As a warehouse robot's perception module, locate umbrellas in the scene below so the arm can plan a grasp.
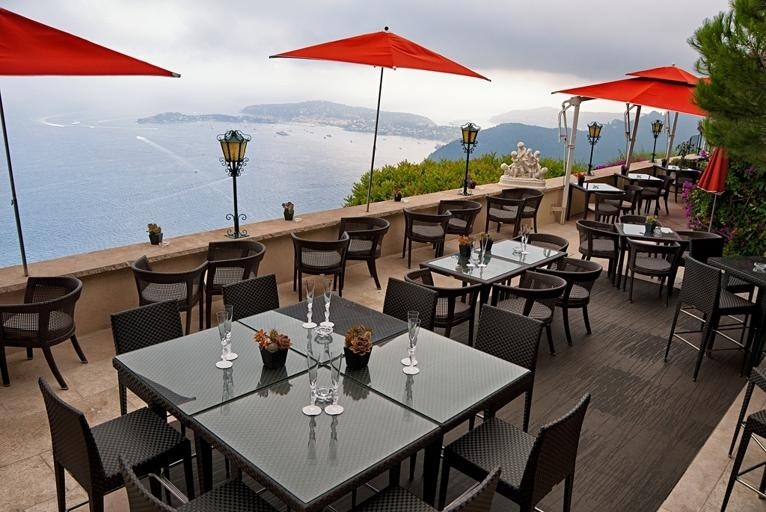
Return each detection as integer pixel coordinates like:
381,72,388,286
266,24,492,212
548,62,733,233
0,7,181,277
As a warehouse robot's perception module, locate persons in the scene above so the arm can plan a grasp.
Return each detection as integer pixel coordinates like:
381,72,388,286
500,141,548,182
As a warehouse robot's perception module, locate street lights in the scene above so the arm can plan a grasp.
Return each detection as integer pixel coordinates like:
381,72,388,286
584,118,604,179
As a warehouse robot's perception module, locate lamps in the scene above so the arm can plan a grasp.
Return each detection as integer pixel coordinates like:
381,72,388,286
583,120,603,175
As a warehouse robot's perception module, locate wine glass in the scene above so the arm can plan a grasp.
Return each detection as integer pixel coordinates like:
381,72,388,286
302,350,322,418
302,278,318,329
326,351,344,416
399,310,418,367
403,318,421,375
320,280,335,328
218,305,238,361
466,234,489,268
520,222,531,255
215,310,233,369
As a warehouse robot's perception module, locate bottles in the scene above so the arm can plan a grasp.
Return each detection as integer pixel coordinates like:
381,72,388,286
653,226,663,238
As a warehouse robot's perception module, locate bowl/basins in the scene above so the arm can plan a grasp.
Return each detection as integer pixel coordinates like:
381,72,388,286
754,262,766,271
